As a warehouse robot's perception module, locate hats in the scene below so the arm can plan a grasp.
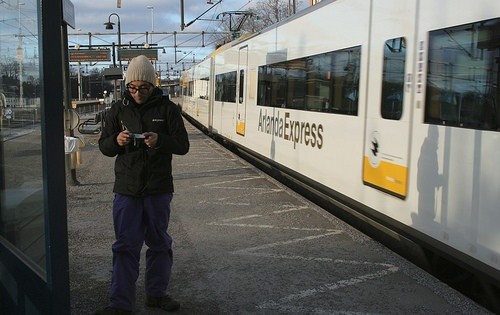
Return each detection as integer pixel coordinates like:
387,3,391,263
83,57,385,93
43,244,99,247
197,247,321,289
125,55,156,90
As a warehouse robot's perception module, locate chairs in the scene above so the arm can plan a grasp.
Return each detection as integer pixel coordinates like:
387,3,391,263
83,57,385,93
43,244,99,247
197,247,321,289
78,107,110,134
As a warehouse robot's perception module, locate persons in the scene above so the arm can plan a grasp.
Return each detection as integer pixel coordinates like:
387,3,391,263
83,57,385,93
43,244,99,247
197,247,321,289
94,55,190,315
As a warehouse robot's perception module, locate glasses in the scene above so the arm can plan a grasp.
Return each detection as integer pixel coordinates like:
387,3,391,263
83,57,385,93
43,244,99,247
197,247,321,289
127,87,151,94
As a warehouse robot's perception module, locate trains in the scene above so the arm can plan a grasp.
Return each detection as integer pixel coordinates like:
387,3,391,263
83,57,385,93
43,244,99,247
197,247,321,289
178,0,500,292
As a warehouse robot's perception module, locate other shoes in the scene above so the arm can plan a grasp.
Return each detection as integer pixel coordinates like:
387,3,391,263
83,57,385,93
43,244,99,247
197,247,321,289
91,304,134,315
145,295,181,311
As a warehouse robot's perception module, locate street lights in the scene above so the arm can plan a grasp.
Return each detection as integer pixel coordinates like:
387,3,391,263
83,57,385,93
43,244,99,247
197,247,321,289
18,2,25,107
145,5,155,45
103,13,124,99
77,28,83,101
